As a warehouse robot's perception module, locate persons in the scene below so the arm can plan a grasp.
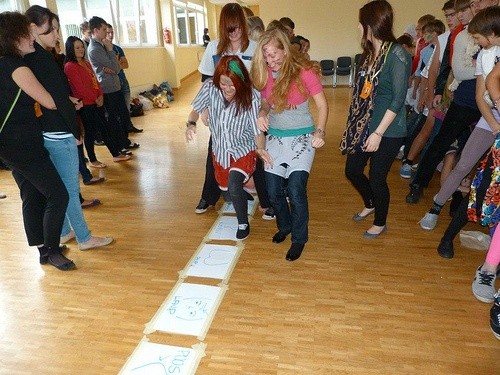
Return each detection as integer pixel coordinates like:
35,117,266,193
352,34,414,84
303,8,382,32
404,0,500,343
243,6,310,56
87,16,140,154
250,29,329,260
106,24,144,132
79,22,92,47
51,41,104,208
338,0,412,239
195,3,276,220
0,11,77,271
64,36,131,168
24,5,113,251
186,55,270,239
202,29,210,46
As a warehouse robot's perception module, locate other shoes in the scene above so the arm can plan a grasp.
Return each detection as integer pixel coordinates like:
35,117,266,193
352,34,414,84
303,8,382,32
113,155,131,161
48,254,75,270
84,178,104,185
128,126,143,133
77,237,112,250
243,189,255,201
262,208,276,220
362,226,386,239
128,143,139,148
352,208,375,221
119,150,132,155
40,244,66,263
438,237,453,258
89,160,106,168
94,139,105,145
406,184,422,203
236,222,250,240
195,199,212,213
419,211,438,230
60,231,74,244
82,200,100,208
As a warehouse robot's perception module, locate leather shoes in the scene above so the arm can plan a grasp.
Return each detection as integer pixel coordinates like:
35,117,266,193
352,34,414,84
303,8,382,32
285,243,304,260
271,231,290,243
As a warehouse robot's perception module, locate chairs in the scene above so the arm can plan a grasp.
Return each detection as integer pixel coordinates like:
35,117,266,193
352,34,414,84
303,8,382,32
320,54,361,87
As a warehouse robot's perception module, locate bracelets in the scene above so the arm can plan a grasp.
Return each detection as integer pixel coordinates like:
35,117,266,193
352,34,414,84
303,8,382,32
374,132,383,137
494,96,500,108
190,121,196,126
319,129,325,135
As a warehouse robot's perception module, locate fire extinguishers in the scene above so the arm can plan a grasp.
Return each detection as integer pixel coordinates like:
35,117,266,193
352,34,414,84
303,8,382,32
163,27,171,43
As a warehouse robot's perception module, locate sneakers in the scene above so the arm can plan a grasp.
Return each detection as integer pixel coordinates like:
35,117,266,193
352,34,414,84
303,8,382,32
398,164,413,178
490,299,500,339
471,265,496,304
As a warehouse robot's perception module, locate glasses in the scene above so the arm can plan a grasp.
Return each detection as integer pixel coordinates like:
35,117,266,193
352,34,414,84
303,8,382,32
445,12,458,19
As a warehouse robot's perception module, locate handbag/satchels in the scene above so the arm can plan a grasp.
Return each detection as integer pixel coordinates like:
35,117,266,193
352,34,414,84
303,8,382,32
129,81,173,117
